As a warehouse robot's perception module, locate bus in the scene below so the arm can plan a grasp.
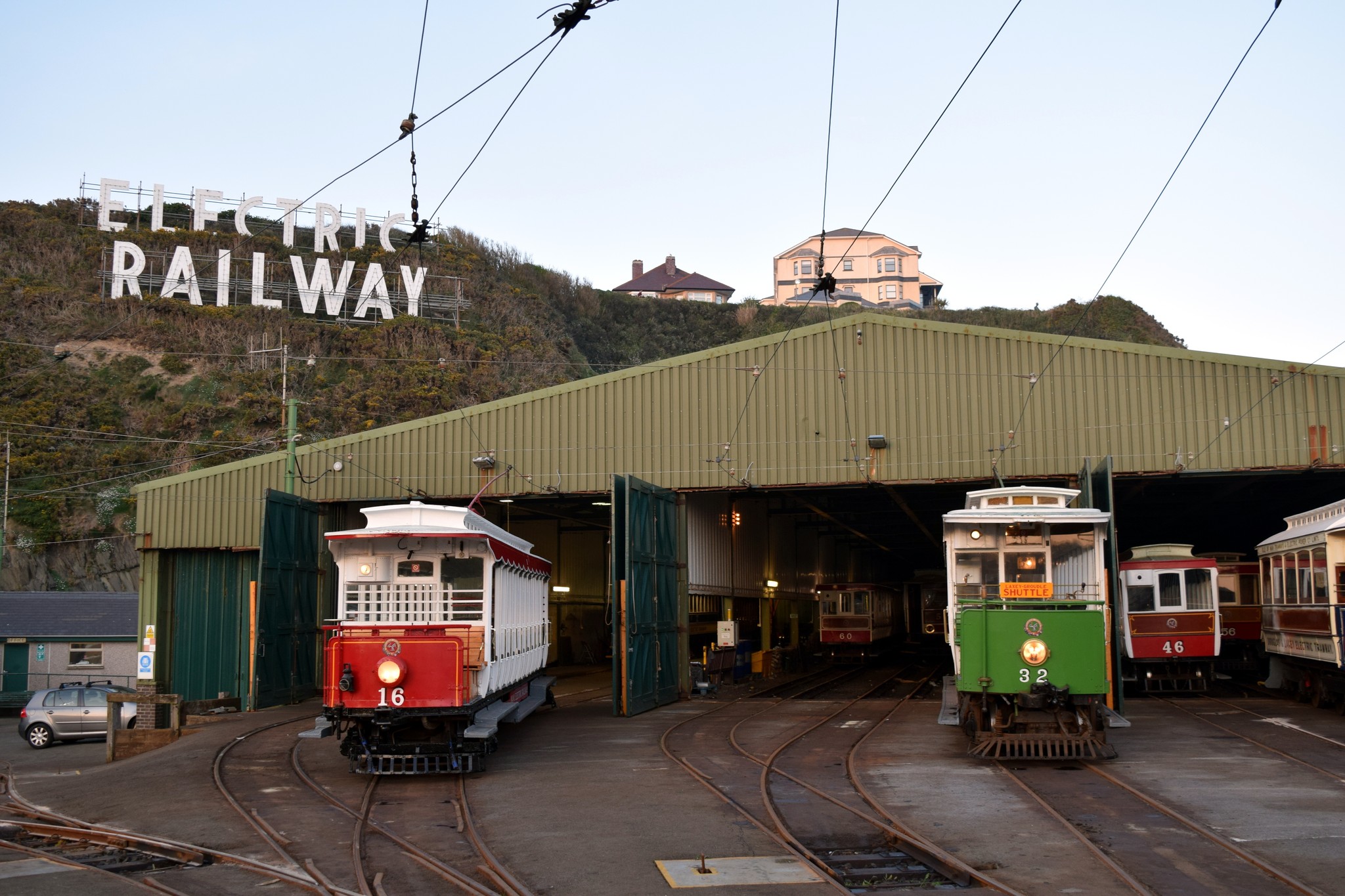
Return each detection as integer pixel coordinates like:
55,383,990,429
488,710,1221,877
937,485,1131,762
813,582,912,657
921,581,948,639
297,465,559,781
1116,498,1345,722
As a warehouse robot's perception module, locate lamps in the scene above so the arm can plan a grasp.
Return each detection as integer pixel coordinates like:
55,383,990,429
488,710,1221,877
766,580,778,587
551,586,570,592
472,456,494,470
868,435,887,448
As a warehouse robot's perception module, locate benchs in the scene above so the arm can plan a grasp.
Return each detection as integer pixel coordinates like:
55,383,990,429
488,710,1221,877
954,612,960,645
703,644,737,688
343,626,488,701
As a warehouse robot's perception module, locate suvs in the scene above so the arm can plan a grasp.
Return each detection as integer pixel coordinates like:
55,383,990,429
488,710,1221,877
17,680,138,749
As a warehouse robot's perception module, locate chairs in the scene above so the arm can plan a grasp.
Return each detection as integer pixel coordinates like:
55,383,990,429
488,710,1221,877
85,693,91,701
89,690,98,698
